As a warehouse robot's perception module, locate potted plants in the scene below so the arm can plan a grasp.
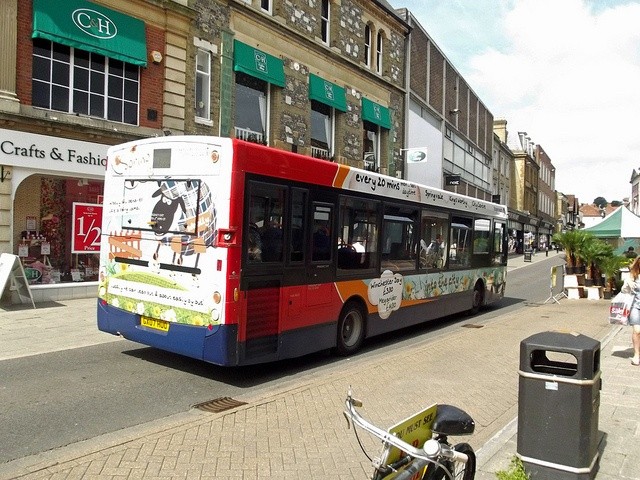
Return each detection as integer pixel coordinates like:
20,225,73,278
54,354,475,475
553,233,575,275
588,241,613,286
601,256,633,300
571,231,587,273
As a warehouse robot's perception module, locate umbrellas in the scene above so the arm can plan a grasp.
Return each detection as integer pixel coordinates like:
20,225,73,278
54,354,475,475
581,205,638,237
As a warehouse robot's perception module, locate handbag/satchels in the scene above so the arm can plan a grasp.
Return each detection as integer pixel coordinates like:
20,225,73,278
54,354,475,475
609,290,634,326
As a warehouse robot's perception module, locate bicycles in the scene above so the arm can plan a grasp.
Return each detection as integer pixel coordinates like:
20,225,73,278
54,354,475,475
340,390,477,480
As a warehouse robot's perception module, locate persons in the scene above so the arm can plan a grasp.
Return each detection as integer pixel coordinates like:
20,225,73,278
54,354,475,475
621,262,640,366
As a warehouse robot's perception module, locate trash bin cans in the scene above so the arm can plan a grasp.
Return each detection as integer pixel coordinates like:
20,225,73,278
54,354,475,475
524,252,532,262
515,332,608,480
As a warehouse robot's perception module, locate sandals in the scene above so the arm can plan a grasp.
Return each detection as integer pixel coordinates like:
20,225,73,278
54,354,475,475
632,357,639,365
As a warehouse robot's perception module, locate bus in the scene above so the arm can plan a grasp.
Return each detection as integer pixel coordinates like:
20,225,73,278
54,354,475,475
96,134,510,368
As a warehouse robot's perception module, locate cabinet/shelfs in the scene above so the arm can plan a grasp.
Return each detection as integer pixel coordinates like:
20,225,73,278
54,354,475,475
20,233,52,271
